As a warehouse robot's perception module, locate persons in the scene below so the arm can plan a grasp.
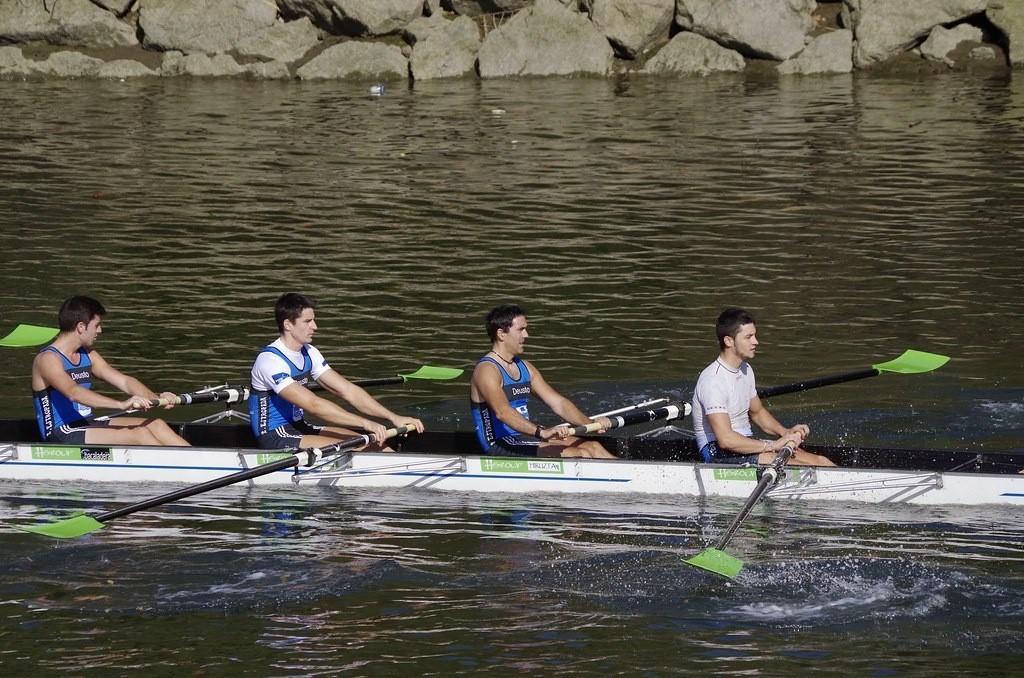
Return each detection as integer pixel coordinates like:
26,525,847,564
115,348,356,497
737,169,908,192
691,308,838,466
31,295,192,446
470,305,621,459
249,293,424,452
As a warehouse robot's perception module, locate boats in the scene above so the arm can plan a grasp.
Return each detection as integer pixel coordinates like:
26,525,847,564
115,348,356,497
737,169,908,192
1,419,1023,511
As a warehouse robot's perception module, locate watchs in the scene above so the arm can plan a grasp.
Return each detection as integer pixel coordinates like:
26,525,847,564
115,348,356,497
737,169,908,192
535,425,546,439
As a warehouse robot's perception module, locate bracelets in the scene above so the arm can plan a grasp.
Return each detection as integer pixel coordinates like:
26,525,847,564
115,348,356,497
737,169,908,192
763,440,768,453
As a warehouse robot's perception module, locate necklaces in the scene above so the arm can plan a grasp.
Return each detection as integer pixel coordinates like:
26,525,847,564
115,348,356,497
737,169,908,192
491,350,513,364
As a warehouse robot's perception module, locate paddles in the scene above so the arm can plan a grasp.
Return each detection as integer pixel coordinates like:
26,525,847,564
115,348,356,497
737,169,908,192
680,428,803,579
568,349,950,437
0,323,61,349
9,423,417,539
133,365,464,412
